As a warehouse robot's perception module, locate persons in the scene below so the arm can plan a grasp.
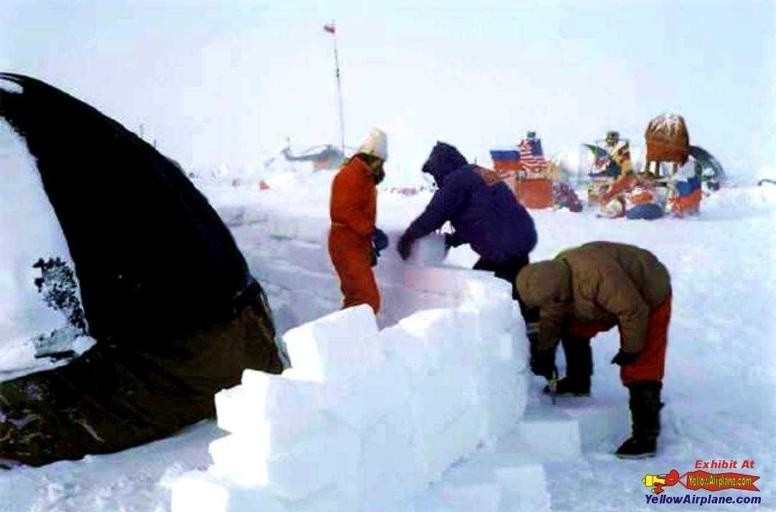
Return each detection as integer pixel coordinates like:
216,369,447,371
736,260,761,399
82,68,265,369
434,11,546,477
396,141,541,355
518,238,673,456
325,127,391,316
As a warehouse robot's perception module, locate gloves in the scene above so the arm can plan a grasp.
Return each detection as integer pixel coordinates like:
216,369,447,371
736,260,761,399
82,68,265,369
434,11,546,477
397,237,413,261
370,229,388,266
541,366,559,381
611,352,635,365
444,233,452,251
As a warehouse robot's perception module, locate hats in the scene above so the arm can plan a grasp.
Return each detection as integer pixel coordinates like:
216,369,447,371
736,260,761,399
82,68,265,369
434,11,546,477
359,129,388,161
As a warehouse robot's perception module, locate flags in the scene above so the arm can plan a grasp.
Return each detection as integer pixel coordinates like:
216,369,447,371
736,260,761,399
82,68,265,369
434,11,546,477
323,23,337,34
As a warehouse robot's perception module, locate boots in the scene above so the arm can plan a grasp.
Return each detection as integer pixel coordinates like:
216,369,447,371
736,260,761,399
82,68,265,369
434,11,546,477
614,380,664,459
543,336,593,397
